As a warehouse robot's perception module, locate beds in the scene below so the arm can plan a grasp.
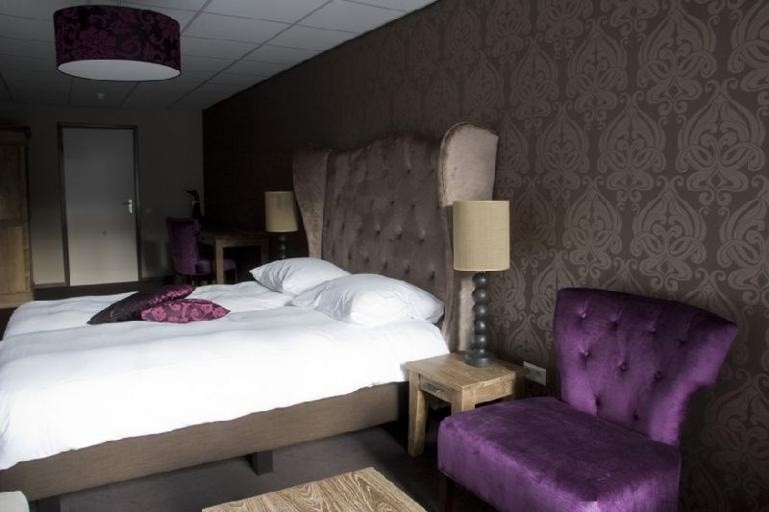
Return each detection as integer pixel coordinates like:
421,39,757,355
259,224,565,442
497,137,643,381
0,119,507,512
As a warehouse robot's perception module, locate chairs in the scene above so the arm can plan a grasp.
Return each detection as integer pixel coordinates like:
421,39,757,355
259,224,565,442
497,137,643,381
436,282,739,511
164,215,237,282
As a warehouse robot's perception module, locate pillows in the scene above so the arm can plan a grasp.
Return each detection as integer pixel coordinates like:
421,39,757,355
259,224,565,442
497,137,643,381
84,279,232,328
247,258,446,337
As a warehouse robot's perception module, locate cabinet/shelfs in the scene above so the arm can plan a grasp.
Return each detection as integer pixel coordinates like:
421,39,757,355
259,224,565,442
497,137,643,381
1,125,40,310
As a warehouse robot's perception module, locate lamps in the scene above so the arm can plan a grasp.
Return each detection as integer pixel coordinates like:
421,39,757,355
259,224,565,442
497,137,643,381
448,198,512,368
53,4,183,83
264,191,303,260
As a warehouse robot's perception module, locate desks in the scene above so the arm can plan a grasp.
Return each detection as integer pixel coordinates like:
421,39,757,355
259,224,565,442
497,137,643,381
195,228,270,283
402,350,522,460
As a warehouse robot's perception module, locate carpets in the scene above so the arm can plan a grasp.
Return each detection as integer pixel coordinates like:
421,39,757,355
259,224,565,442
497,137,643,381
201,466,429,512
